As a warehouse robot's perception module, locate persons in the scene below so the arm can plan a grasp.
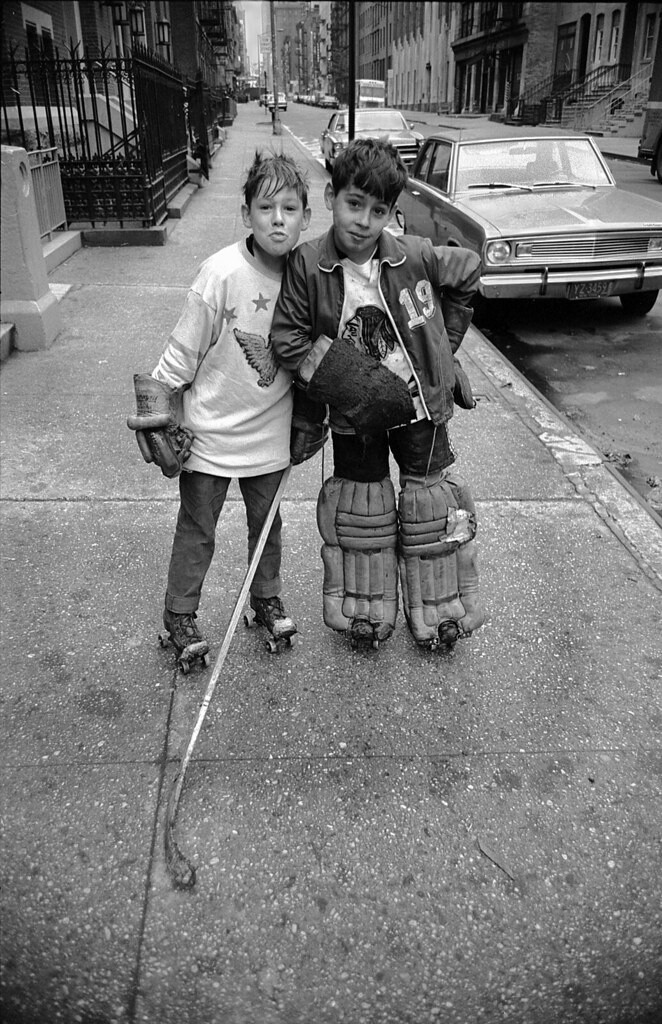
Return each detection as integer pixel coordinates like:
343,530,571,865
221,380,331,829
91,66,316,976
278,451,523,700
126,151,331,674
269,139,485,652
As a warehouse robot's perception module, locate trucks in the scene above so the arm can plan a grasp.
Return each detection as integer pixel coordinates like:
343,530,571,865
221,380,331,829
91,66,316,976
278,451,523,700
354,78,387,109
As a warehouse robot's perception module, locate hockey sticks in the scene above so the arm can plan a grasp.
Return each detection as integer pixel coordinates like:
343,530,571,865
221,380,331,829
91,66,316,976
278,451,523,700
163,460,295,889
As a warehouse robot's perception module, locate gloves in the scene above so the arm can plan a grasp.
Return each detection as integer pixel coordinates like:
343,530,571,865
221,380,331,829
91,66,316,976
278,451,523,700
304,339,417,439
127,373,194,479
440,297,478,410
290,379,329,466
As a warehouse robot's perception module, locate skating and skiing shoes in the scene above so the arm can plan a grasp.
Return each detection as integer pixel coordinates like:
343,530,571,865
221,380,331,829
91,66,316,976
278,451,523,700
428,621,459,651
351,619,380,650
243,594,296,654
158,608,210,675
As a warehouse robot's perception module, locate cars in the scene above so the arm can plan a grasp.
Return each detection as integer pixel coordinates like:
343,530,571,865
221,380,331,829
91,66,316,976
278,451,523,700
394,125,662,323
291,94,340,110
318,109,426,176
259,91,288,112
